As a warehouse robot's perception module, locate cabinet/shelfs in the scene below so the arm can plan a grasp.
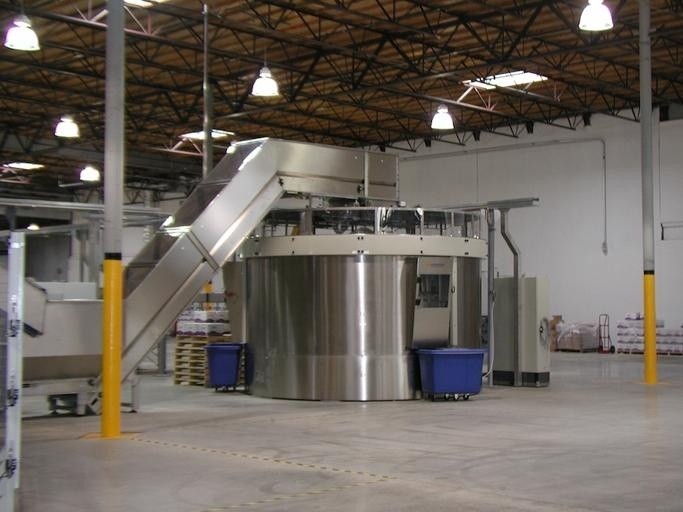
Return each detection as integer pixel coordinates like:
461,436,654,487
173,335,231,388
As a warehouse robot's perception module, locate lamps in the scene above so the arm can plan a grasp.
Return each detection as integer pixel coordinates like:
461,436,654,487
4,0,40,51
431,104,454,130
80,166,101,182
578,0,614,32
251,0,278,97
54,114,80,138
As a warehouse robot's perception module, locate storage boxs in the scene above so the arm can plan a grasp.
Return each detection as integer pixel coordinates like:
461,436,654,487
617,313,683,353
416,348,486,401
175,302,231,336
204,342,242,393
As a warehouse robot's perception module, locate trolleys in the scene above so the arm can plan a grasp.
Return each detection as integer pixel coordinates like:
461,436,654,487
598,313,615,353
